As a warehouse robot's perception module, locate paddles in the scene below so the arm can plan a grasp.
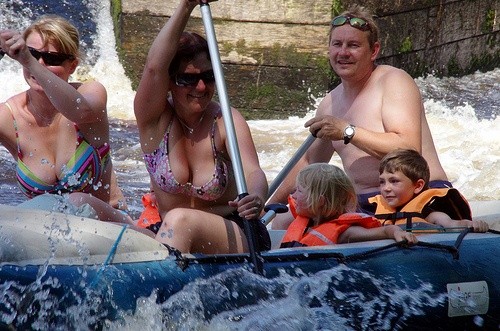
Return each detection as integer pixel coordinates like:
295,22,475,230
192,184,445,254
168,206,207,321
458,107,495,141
264,132,317,206
200,0,264,278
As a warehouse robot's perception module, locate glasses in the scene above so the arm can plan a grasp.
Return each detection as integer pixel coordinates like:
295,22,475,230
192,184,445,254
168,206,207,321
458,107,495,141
331,16,374,34
170,69,215,86
28,46,75,66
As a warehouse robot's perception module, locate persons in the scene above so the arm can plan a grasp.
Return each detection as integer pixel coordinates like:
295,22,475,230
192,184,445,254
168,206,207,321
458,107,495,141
262,7,489,248
134,0,271,254
0,14,134,224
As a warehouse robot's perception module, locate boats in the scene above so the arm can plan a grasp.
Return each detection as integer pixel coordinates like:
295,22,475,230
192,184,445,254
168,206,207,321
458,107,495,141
0,202,500,331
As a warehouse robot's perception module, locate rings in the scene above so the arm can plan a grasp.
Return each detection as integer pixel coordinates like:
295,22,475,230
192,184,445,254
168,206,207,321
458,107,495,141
254,207,259,212
12,38,17,42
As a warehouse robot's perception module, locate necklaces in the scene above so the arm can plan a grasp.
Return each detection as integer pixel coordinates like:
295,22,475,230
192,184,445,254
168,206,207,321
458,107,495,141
27,91,58,127
175,112,206,135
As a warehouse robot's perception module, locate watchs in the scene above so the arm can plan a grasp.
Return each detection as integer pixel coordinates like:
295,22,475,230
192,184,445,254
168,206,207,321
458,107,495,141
343,124,356,145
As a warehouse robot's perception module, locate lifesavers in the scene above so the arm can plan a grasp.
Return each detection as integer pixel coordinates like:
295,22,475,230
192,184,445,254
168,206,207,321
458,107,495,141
0,204,168,263
